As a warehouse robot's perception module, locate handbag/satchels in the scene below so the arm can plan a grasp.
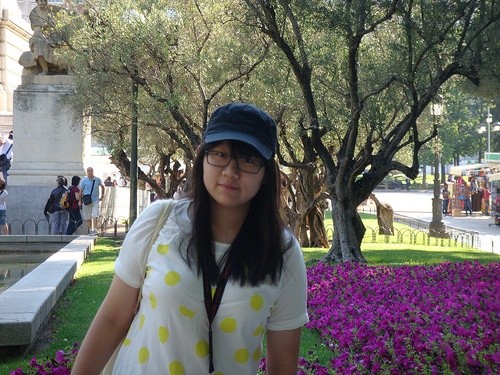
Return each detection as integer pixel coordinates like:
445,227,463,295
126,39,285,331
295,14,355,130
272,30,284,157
82,194,92,205
0,154,7,167
46,199,55,214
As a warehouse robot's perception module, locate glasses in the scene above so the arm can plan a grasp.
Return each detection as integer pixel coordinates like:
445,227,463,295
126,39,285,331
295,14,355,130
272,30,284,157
202,149,265,175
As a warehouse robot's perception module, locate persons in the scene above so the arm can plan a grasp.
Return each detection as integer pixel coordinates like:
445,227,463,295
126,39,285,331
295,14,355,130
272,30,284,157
462,182,474,216
442,183,450,214
484,186,492,216
148,167,187,203
0,130,13,235
72,104,309,375
45,167,105,235
104,177,113,187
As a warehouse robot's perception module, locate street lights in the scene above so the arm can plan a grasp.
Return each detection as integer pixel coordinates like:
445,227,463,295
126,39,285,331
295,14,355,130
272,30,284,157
430,94,451,239
486,106,494,153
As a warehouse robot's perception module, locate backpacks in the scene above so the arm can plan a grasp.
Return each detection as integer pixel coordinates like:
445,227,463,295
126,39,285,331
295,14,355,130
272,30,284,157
59,185,77,210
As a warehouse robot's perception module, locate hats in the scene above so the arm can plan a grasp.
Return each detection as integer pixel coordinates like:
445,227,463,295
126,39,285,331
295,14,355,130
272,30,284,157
202,102,276,160
